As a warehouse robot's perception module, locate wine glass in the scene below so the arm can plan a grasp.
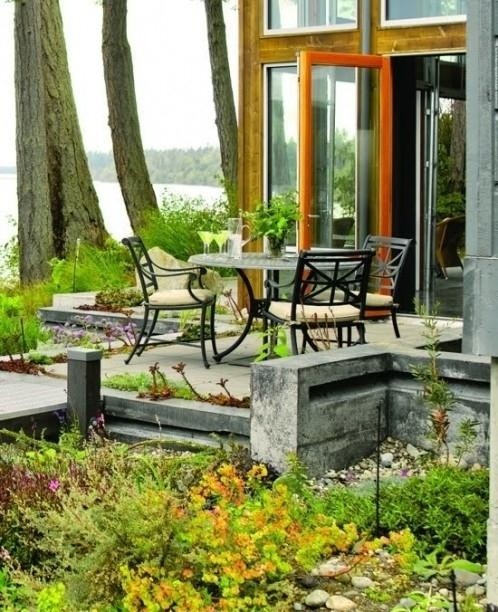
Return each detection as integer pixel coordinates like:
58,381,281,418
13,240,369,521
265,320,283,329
197,229,229,260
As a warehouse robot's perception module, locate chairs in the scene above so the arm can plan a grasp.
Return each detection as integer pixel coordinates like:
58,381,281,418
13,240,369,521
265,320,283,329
306,211,417,342
120,231,223,370
330,217,355,247
429,213,467,283
261,247,380,361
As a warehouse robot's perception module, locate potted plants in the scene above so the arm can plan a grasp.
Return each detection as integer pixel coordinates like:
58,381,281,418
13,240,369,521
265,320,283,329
237,189,304,259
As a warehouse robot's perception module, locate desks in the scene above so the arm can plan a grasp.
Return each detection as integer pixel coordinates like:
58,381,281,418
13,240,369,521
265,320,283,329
187,239,369,365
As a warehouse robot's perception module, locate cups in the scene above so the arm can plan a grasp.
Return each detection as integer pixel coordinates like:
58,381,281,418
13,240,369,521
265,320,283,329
228,216,250,260
